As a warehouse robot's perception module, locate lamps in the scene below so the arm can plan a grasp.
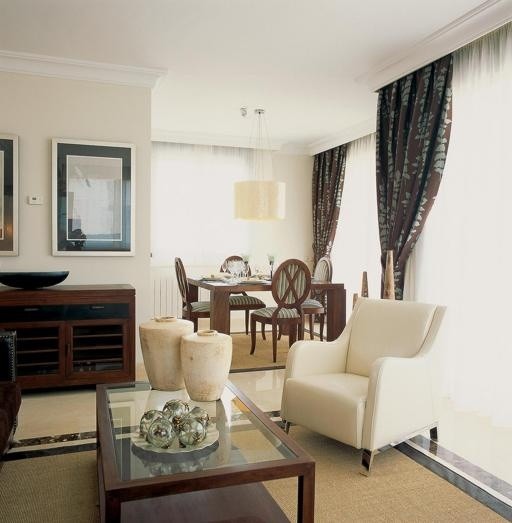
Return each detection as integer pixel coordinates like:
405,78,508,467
234,108,286,221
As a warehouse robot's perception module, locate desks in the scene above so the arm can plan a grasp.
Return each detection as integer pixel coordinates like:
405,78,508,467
186,275,346,342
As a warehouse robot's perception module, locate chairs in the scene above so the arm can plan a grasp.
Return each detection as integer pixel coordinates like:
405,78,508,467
220,256,266,340
175,257,210,332
278,257,333,342
250,259,311,363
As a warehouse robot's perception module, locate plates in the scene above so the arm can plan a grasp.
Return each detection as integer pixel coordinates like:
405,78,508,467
130,422,219,453
0,271,69,288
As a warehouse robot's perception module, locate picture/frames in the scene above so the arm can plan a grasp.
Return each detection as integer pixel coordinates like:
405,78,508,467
52,138,136,257
0,133,19,256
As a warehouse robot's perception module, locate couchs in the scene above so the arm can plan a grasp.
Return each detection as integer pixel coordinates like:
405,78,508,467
0,331,22,470
280,297,447,478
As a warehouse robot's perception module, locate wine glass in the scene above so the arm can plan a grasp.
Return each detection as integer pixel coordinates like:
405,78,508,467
227,261,249,282
222,261,227,272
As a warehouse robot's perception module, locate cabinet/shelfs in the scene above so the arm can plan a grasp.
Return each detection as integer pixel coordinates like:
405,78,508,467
0,285,136,389
